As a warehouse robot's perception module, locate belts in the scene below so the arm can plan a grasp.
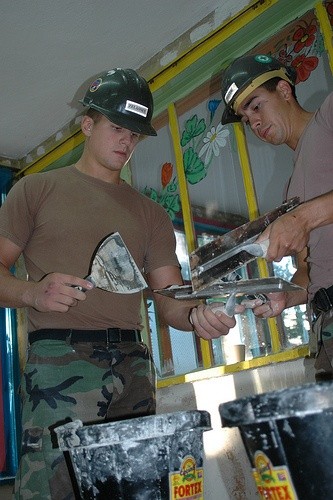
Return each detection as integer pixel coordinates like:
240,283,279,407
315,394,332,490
308,285,333,322
27,327,141,345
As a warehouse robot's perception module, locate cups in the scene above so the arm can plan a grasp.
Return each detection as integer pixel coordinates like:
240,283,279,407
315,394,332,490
251,347,266,358
229,345,244,363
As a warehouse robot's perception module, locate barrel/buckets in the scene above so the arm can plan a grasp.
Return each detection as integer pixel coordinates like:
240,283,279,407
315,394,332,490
219,381,333,500
54,408,213,500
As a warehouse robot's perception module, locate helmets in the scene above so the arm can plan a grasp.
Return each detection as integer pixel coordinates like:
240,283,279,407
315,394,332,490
220,54,297,125
76,67,157,137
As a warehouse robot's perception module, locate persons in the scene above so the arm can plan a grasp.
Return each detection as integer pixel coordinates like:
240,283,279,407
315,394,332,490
222,57,333,381
0,68,236,500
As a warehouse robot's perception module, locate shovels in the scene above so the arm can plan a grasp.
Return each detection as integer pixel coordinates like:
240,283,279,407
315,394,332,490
153,276,304,324
191,196,300,292
73,232,149,294
